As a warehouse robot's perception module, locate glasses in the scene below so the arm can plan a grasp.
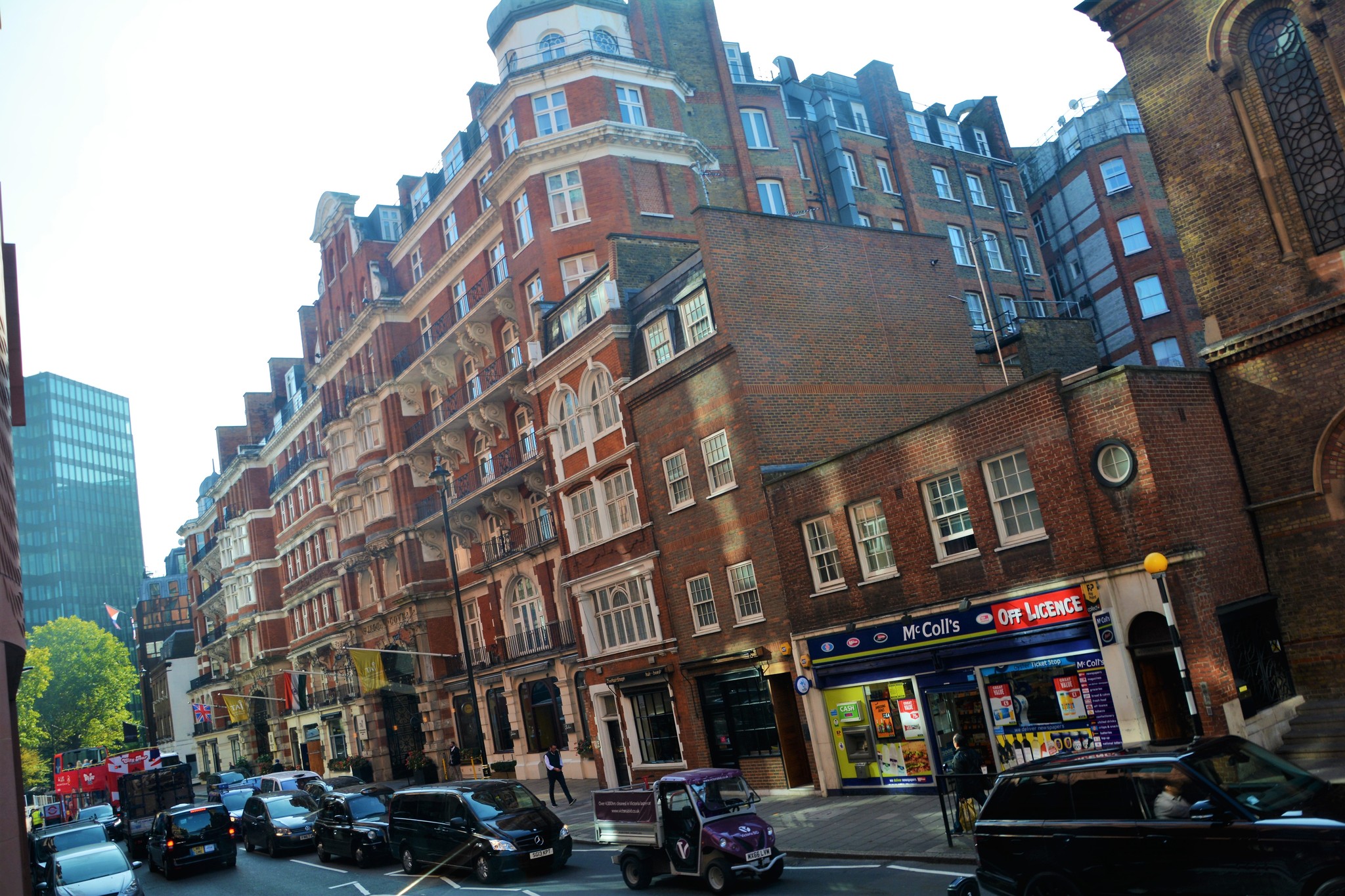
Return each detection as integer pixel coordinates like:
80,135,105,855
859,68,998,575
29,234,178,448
551,747,557,750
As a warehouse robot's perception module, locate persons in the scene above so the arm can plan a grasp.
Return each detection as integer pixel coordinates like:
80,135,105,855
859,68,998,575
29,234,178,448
1154,772,1196,823
28,809,38,830
65,790,106,823
66,756,108,771
447,741,464,782
943,732,989,838
228,762,235,770
271,759,284,774
543,741,577,808
32,805,45,832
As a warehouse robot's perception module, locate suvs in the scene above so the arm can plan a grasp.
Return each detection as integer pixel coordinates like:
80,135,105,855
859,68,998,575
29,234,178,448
72,802,124,842
970,737,1344,895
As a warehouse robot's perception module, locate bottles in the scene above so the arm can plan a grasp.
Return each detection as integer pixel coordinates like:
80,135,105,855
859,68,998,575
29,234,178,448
876,717,893,733
1059,693,1076,715
994,731,1046,771
877,742,905,776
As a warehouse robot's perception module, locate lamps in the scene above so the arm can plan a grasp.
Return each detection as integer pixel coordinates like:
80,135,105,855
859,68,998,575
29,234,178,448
846,621,856,634
901,614,912,626
959,600,972,613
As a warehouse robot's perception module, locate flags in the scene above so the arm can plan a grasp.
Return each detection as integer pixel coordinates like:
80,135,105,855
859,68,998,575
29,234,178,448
222,695,250,724
105,604,122,630
283,670,309,712
121,721,139,744
131,619,137,640
346,648,391,694
192,704,214,724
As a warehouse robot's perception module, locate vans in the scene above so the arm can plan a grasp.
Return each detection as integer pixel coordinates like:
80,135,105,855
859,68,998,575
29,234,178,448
389,778,573,882
28,816,116,896
260,770,323,794
36,841,143,896
206,767,256,796
143,801,237,878
207,785,265,839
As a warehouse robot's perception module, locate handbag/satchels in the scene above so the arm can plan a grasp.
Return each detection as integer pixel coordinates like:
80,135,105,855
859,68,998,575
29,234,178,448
977,768,994,790
959,798,978,832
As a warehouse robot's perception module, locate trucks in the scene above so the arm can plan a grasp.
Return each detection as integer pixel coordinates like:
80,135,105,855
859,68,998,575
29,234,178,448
113,763,195,859
591,766,787,892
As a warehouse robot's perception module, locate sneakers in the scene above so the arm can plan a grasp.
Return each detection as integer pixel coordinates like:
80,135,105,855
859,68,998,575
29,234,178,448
552,804,559,807
570,798,577,805
945,829,964,837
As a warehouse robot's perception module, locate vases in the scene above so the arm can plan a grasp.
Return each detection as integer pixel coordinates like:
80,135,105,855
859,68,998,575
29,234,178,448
351,764,373,785
582,751,594,758
412,764,439,786
459,757,479,765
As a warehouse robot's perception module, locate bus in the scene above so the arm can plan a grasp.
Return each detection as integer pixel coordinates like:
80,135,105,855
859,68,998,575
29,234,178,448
54,745,163,826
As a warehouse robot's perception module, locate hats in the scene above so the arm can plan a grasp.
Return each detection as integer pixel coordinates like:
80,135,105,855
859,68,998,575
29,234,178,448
275,759,279,763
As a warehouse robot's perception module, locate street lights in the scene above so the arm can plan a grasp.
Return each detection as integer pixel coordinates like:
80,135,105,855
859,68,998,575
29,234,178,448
428,455,494,780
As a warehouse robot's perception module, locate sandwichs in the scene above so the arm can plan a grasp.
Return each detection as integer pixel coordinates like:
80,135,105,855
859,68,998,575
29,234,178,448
901,741,931,775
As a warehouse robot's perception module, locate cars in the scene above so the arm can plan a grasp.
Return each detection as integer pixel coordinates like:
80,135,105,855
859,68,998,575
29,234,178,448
314,783,397,866
305,775,367,804
241,790,321,858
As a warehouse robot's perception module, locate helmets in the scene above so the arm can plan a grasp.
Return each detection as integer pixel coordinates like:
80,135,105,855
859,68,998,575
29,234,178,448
34,805,40,810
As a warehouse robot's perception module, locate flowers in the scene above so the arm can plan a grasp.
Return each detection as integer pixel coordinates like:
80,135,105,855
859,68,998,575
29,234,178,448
346,755,370,768
458,748,478,759
259,763,303,774
328,758,351,771
573,738,592,756
405,750,434,770
